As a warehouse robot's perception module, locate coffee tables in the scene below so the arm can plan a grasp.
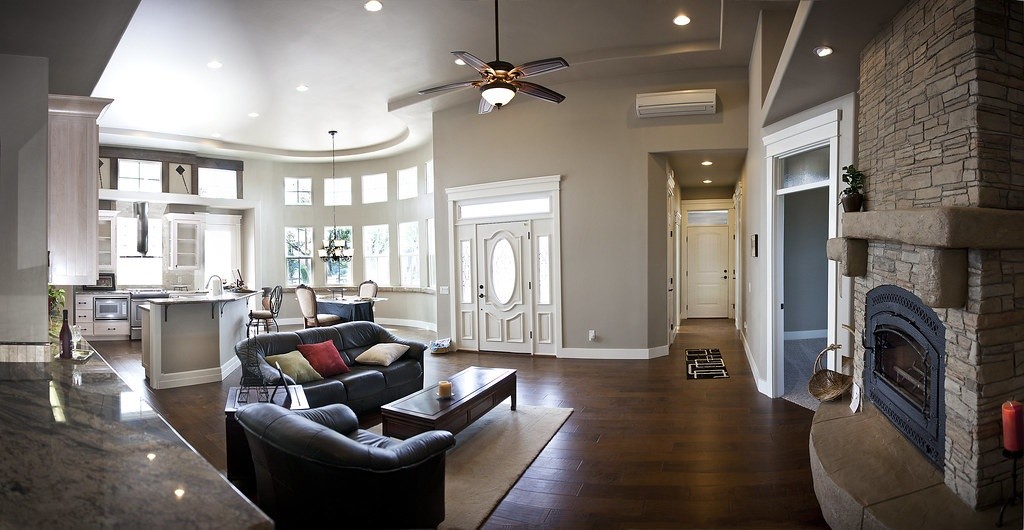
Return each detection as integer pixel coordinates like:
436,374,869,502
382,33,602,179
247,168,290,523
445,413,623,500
380,367,516,440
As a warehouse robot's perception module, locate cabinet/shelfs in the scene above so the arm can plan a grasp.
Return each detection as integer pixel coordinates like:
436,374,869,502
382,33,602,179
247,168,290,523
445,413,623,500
76,295,147,342
165,212,202,271
98,210,120,269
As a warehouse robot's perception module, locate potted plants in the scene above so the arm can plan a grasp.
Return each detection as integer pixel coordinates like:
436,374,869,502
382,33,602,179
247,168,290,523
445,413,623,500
838,165,865,212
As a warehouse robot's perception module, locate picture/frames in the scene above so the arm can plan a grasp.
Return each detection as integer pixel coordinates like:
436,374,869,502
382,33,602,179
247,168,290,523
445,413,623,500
84,272,115,290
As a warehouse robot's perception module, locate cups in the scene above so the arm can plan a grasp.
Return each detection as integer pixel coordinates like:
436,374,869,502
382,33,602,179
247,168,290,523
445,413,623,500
439,380,451,396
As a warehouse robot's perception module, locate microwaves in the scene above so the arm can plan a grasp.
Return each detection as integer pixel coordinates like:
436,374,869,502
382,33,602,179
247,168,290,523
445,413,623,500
83,273,115,291
96,299,127,319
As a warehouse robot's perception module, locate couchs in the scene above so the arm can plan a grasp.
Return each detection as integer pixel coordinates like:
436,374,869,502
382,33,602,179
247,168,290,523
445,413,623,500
235,402,456,530
236,321,427,423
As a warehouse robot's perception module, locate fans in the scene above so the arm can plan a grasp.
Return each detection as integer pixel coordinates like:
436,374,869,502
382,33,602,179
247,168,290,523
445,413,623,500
419,0,571,114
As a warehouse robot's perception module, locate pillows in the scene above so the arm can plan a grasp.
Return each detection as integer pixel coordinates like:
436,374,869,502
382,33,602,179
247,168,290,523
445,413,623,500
262,352,325,383
295,339,351,378
355,343,410,366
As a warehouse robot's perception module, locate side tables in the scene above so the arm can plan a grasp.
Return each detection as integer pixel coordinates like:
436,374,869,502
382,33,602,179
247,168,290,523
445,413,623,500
225,384,308,483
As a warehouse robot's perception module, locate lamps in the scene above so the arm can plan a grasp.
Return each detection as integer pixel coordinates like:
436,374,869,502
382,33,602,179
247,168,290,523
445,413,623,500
479,83,517,111
319,130,355,262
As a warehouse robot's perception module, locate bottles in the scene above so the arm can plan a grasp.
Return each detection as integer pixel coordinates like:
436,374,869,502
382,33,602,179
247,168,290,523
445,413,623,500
59,309,72,359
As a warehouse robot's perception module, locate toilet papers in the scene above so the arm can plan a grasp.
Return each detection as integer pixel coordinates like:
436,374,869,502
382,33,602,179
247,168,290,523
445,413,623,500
211,279,220,297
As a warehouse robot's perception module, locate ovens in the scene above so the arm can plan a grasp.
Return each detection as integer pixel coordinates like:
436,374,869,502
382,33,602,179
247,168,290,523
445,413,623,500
130,298,150,339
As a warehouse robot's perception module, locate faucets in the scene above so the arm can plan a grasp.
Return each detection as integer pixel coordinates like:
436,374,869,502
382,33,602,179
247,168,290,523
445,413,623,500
205,275,223,298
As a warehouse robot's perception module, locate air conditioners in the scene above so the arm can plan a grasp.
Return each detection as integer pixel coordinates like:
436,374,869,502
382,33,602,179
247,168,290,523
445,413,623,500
637,87,718,117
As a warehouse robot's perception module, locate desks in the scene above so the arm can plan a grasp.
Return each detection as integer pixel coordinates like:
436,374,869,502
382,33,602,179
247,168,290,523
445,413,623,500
0,331,276,530
295,294,375,323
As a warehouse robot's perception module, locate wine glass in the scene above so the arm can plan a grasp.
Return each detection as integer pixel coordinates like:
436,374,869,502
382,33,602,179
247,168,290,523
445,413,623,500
70,325,81,353
72,363,82,386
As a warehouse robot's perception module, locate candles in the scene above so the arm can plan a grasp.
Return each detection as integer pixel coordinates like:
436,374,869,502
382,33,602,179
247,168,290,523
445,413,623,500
439,380,451,397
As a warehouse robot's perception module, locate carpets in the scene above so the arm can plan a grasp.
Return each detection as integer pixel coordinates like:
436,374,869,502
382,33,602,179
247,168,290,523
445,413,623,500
685,347,730,379
367,408,573,529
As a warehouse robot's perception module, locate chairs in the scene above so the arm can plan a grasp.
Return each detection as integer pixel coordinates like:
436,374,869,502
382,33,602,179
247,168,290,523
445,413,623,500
246,285,283,337
358,280,379,306
296,284,341,329
